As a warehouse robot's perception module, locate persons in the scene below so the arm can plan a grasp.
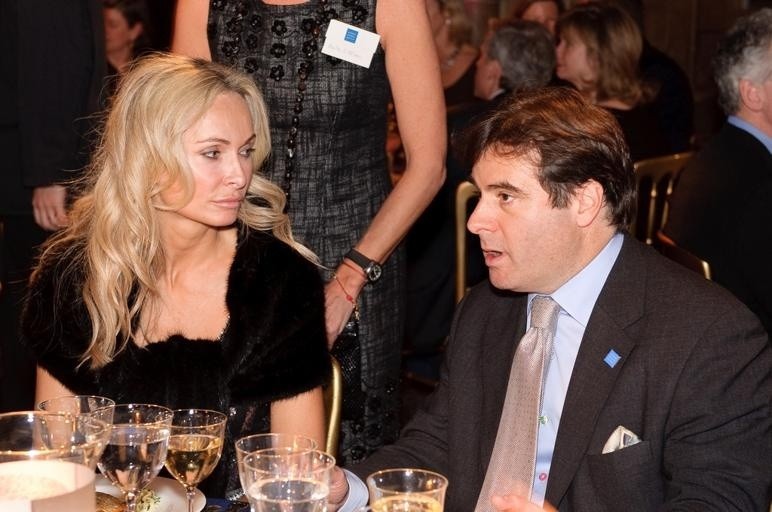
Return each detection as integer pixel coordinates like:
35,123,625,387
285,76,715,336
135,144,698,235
649,7,772,337
385,1,694,424
174,0,447,464
20,51,332,502
334,84,771,512
2,2,155,443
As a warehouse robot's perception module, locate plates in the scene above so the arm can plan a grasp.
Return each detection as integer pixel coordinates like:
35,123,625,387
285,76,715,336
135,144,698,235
94,473,207,511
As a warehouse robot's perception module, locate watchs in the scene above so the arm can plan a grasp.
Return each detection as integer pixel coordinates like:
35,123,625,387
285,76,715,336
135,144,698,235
344,249,382,282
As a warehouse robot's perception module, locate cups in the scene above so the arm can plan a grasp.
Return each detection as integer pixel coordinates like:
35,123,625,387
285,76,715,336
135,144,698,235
366,467,450,511
0,395,115,512
235,433,336,512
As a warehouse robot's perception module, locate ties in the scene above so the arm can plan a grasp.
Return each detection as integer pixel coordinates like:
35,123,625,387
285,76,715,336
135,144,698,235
474,295,562,512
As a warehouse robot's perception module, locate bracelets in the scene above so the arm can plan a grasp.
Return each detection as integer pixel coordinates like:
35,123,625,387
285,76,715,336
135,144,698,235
333,274,360,320
341,261,367,279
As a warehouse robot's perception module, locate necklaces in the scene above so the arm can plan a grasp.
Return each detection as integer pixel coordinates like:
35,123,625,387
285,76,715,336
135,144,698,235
232,0,324,214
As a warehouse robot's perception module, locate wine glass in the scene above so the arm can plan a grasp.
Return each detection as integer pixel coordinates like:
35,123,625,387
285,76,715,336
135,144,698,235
95,404,228,512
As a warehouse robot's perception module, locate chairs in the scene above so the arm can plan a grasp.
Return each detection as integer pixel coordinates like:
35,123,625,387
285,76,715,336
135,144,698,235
627,150,715,283
452,175,482,319
